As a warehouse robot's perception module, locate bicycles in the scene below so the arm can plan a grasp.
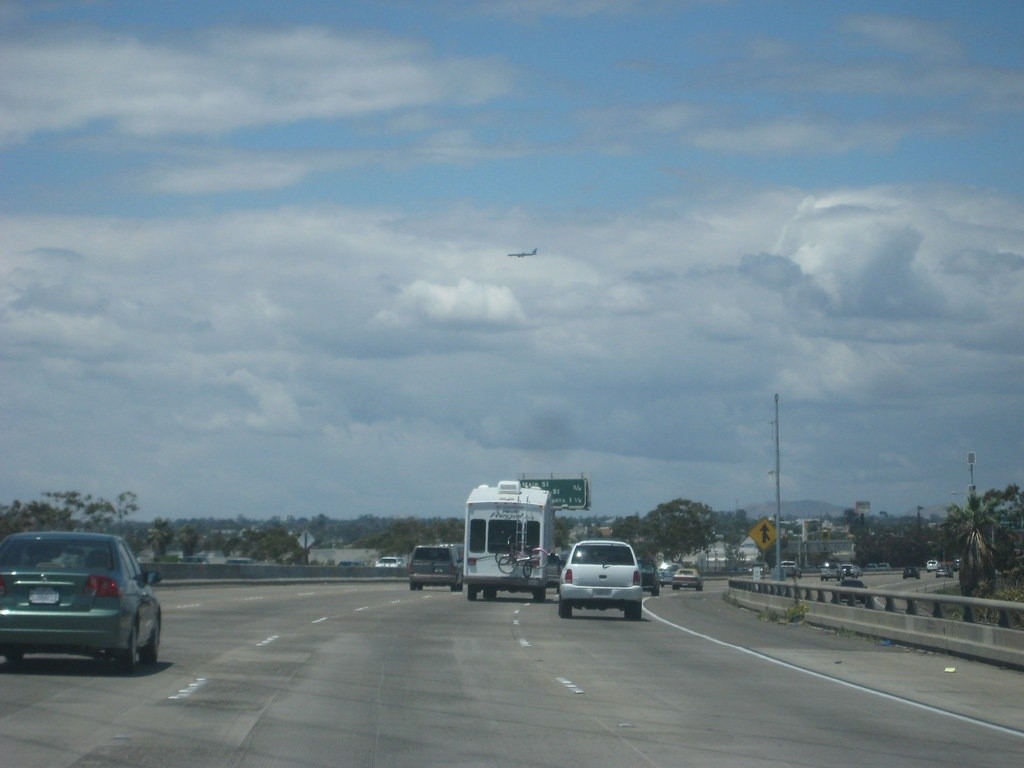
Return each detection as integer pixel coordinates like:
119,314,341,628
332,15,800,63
495,535,550,577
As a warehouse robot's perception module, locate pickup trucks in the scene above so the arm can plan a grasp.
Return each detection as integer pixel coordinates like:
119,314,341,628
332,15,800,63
780,560,802,579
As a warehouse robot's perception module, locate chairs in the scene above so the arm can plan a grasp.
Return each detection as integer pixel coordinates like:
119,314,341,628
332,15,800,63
88,549,107,567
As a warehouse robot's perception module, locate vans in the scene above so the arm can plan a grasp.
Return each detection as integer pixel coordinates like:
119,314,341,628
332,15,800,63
546,553,563,586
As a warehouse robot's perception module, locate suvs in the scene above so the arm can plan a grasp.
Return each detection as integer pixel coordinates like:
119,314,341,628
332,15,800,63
926,559,942,573
407,544,464,592
660,563,684,587
820,562,845,582
637,558,660,597
374,557,407,569
558,540,644,620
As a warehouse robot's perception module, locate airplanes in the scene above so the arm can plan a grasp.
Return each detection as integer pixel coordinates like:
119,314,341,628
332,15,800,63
507,248,538,259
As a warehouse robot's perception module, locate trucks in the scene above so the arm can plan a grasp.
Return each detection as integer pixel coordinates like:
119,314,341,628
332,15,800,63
461,480,557,604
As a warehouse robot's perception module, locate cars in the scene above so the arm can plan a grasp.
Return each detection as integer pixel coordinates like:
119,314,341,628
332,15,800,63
865,563,892,569
936,566,953,578
842,564,864,579
903,566,920,579
181,556,209,565
747,562,769,576
672,569,703,591
0,532,161,673
953,559,960,572
224,557,257,565
338,561,365,567
